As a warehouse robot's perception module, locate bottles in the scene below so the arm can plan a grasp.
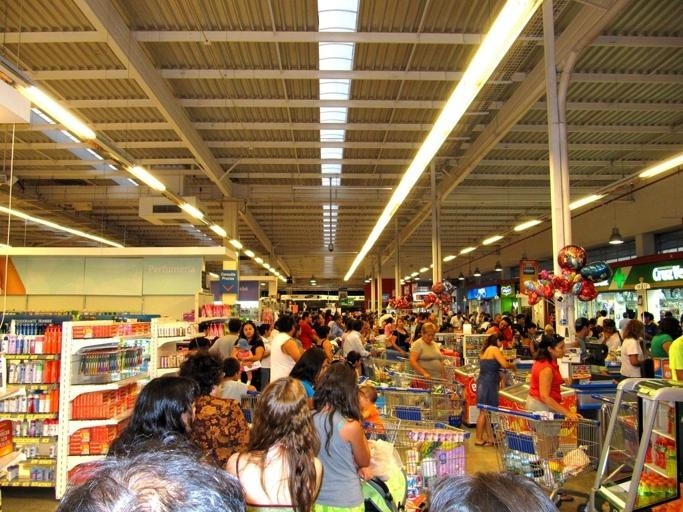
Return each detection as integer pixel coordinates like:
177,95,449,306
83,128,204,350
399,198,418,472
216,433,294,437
504,447,531,479
631,402,678,506
157,327,191,367
199,303,233,337
1,318,61,482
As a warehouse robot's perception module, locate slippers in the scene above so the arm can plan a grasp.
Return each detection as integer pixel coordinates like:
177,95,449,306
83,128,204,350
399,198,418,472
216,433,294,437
474,439,501,447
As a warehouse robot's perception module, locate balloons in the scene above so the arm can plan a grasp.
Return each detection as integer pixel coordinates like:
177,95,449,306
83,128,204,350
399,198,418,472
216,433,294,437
389,295,413,310
523,245,612,304
424,281,455,309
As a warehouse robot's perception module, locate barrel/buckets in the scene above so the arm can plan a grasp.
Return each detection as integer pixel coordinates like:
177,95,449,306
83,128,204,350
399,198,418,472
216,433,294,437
432,441,465,477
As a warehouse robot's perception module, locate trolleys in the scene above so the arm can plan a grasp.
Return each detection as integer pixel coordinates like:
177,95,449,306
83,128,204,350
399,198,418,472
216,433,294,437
588,393,641,489
476,403,603,512
238,391,262,428
498,369,519,387
354,349,471,512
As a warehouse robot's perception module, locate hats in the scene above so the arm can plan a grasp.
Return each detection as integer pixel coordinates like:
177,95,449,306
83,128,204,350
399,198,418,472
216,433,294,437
233,338,252,351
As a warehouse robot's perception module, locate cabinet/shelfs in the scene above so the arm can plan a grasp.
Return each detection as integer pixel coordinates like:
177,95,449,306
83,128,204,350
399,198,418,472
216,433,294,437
588,379,683,512
66,319,149,500
151,316,205,377
0,324,65,499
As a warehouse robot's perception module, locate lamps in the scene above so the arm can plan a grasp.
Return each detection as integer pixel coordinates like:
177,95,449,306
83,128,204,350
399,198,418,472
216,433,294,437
229,239,243,250
244,250,287,282
208,224,227,237
401,153,683,283
128,166,166,191
18,85,96,139
179,203,203,218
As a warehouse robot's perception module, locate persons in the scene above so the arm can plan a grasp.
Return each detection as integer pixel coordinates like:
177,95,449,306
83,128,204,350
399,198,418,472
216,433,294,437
429,472,559,512
290,312,386,442
573,311,682,384
410,322,446,387
226,379,323,512
180,351,251,468
58,432,248,511
190,319,271,406
107,376,213,465
53,309,683,512
309,362,372,512
270,316,301,383
528,334,584,501
381,312,553,359
474,333,514,446
